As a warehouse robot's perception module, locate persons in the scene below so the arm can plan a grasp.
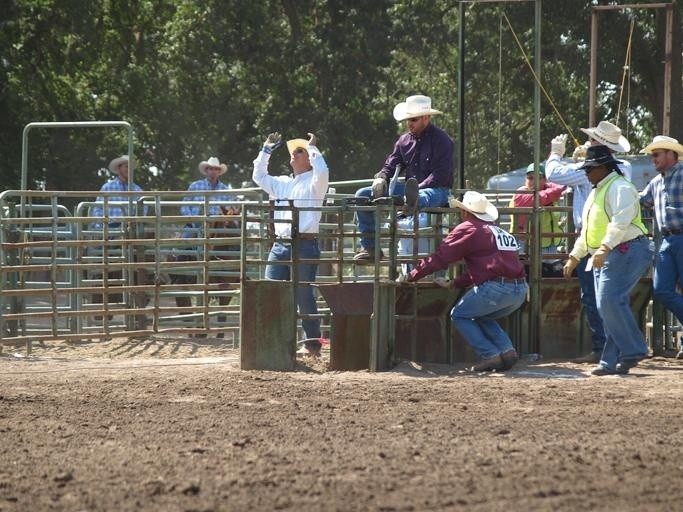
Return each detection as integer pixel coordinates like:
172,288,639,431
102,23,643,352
638,137,683,358
563,148,657,377
509,163,570,270
253,131,329,357
92,155,148,261
353,95,455,261
405,190,528,370
544,121,633,363
571,144,590,162
175,157,241,286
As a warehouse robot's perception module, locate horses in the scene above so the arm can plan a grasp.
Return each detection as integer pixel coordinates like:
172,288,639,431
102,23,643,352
167,206,242,338
82,222,154,320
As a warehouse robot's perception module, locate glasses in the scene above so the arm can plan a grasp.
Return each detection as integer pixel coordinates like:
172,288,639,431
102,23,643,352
652,152,662,157
295,146,306,153
584,167,593,172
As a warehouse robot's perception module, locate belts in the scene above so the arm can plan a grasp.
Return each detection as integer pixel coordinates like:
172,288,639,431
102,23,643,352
629,235,647,241
489,275,526,285
276,233,316,246
664,229,682,236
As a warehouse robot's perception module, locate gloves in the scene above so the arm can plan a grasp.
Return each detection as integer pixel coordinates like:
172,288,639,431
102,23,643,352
563,255,579,279
371,178,387,198
307,133,318,147
592,244,609,268
550,134,567,158
573,145,588,160
433,277,456,293
262,132,281,155
396,272,412,284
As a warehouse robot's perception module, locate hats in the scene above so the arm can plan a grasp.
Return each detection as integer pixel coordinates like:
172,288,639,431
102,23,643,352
393,94,443,123
526,163,545,175
287,139,310,157
640,136,683,159
451,191,498,222
580,122,630,153
199,156,228,176
109,154,128,174
575,145,623,170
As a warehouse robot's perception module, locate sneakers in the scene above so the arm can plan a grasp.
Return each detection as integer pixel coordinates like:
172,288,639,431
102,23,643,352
352,246,384,259
402,177,420,216
574,351,599,363
591,352,649,375
296,346,321,357
474,350,518,372
